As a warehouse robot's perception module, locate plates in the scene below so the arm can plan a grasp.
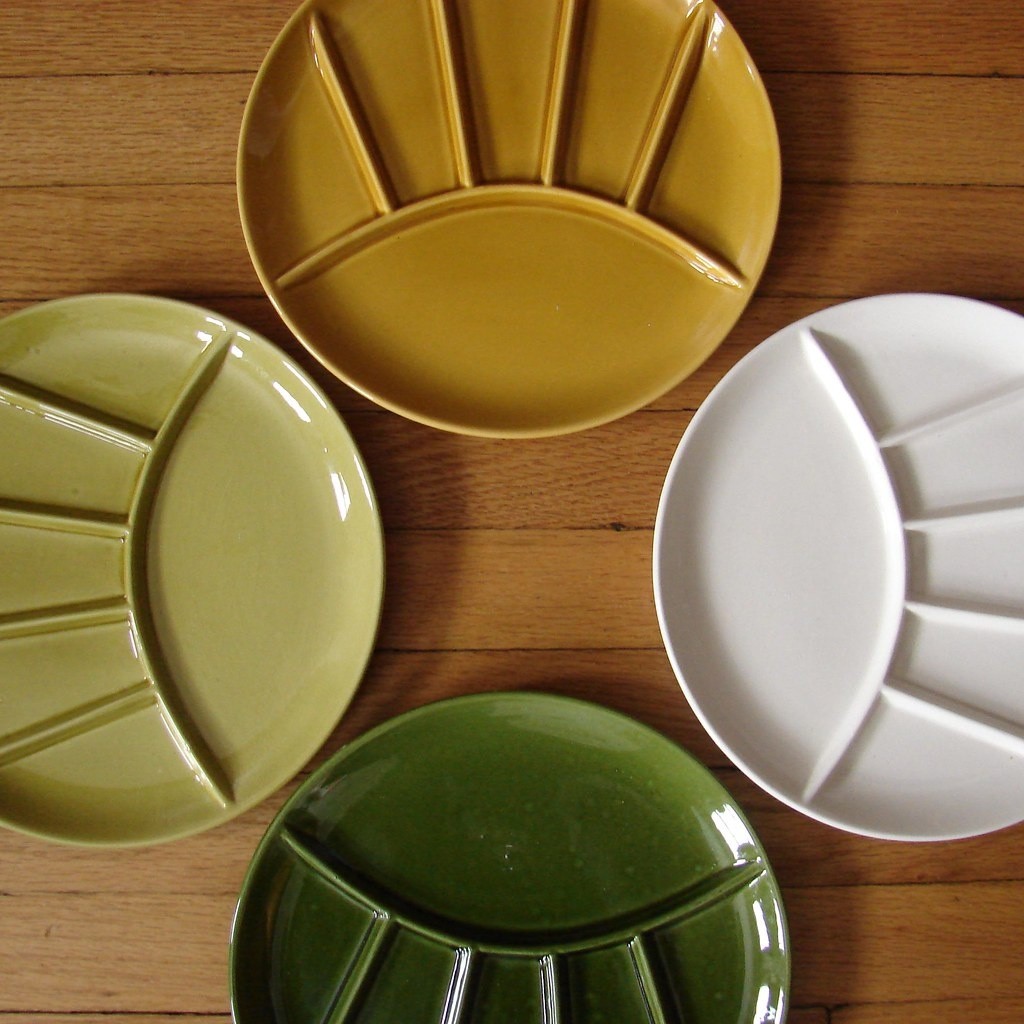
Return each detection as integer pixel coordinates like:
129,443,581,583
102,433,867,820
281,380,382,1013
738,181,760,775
652,290,1022,843
228,693,791,1024
1,291,384,847
235,0,781,439
